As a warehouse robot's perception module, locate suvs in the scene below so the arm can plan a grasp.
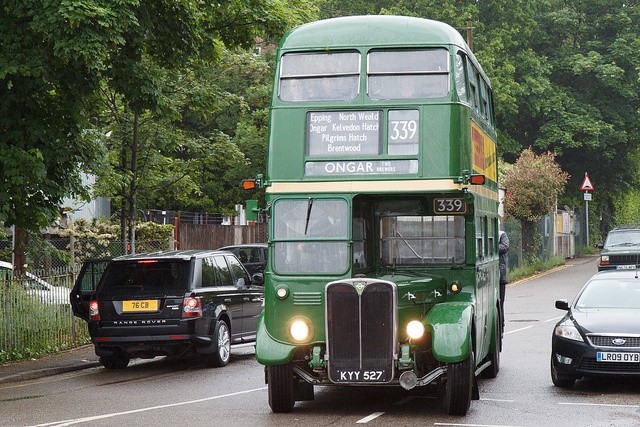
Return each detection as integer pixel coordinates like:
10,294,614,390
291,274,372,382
598,226,640,269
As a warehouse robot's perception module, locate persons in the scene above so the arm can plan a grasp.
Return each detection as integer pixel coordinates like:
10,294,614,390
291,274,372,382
499,229,509,340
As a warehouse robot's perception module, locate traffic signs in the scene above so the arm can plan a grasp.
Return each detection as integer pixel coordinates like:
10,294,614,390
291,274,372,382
579,174,594,194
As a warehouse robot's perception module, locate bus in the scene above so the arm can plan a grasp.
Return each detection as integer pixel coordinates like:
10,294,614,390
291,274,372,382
254,16,510,416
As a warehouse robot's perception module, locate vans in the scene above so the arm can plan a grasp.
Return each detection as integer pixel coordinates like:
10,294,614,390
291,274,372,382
70,248,263,365
216,244,269,278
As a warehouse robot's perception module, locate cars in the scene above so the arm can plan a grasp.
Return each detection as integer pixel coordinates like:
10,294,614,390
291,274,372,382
0,257,70,310
550,268,640,388
